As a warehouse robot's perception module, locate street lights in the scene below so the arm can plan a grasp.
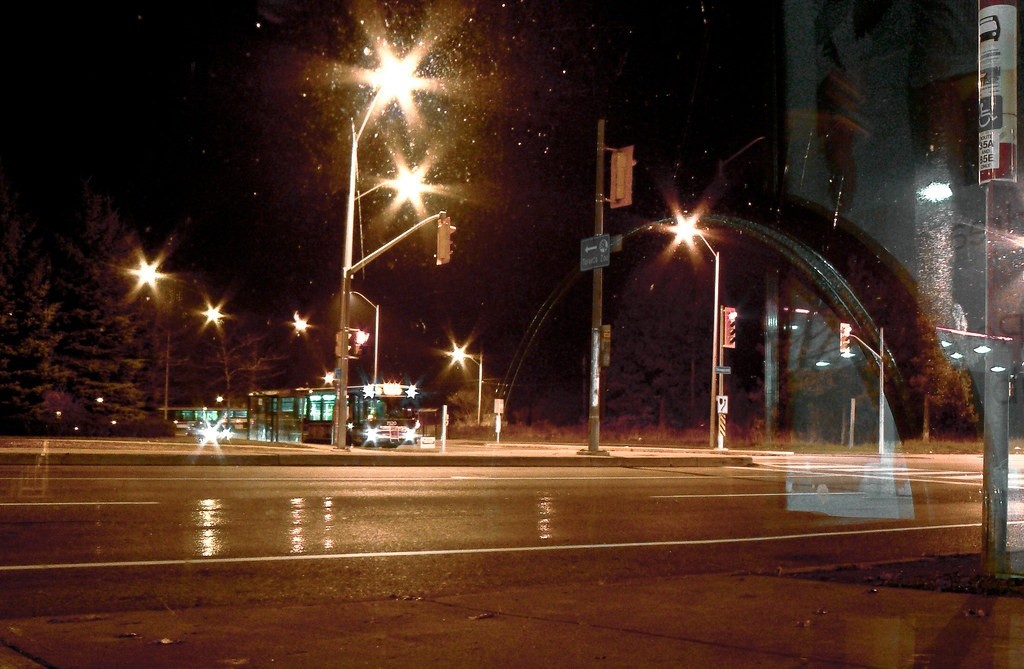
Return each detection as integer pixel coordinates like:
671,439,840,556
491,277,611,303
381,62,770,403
673,220,721,449
139,263,171,419
330,52,422,449
450,349,483,427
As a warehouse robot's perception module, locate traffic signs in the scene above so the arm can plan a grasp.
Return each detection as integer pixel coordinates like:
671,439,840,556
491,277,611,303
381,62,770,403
580,234,611,271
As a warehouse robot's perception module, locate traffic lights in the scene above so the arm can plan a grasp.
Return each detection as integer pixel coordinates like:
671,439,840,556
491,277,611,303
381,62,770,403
350,329,367,358
610,146,638,208
724,306,737,349
840,323,852,354
435,218,457,267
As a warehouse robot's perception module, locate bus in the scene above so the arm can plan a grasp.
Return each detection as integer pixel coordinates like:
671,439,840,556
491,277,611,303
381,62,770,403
248,384,419,451
